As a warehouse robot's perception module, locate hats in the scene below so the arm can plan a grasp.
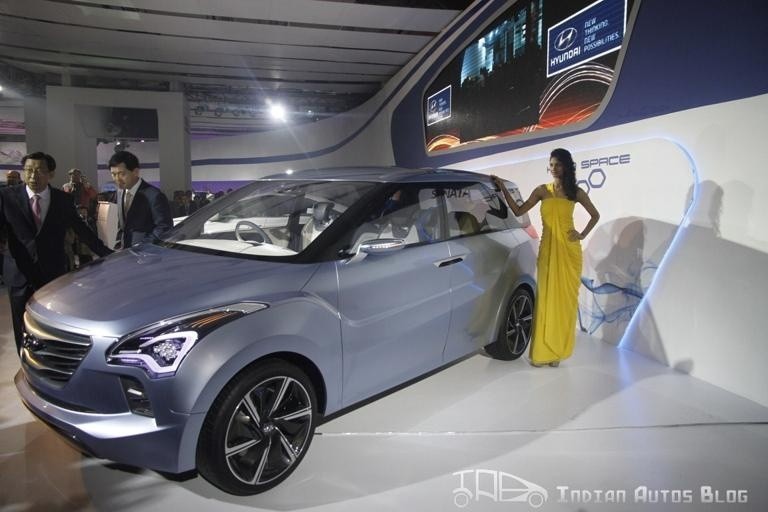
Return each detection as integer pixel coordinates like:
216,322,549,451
68,168,80,174
76,205,88,209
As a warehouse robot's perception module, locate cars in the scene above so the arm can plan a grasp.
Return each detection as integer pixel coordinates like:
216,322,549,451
171,187,351,235
15,165,540,496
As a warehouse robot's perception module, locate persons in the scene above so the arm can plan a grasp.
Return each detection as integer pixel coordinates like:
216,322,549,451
62,168,98,271
109,151,173,250
0,170,25,187
169,191,224,218
0,152,116,358
492,149,600,367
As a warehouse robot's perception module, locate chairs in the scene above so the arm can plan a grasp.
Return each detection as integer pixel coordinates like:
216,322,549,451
298,201,480,255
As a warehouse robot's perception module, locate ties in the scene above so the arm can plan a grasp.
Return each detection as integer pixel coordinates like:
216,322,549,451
124,191,131,217
31,196,41,231
